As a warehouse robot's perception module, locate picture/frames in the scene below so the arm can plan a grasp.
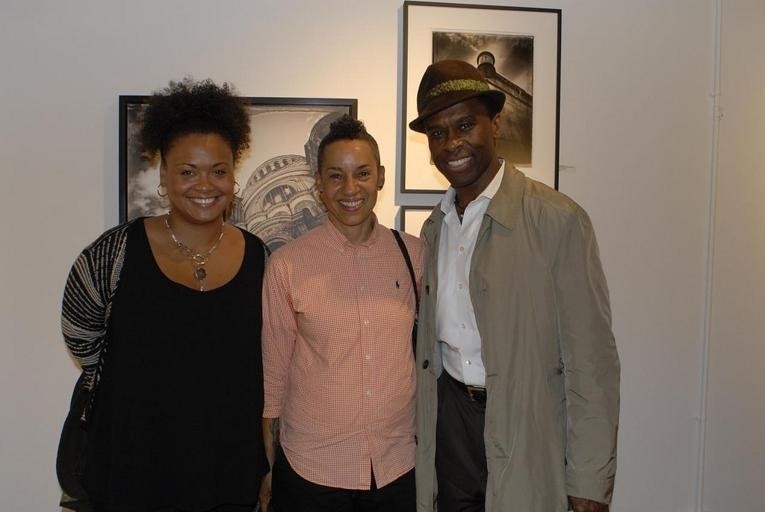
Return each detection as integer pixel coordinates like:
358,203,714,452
399,205,435,238
119,94,359,252
400,0,562,194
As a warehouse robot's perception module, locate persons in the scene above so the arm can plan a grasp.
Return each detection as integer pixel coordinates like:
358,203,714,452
409,62,622,512
262,112,425,510
55,78,274,510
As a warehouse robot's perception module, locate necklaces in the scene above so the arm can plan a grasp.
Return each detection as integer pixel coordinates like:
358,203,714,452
165,211,224,292
456,201,464,222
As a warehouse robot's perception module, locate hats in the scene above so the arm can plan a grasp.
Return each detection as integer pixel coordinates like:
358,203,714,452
410,59,505,131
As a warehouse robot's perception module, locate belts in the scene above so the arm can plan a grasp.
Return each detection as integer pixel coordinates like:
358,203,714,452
450,378,485,403
